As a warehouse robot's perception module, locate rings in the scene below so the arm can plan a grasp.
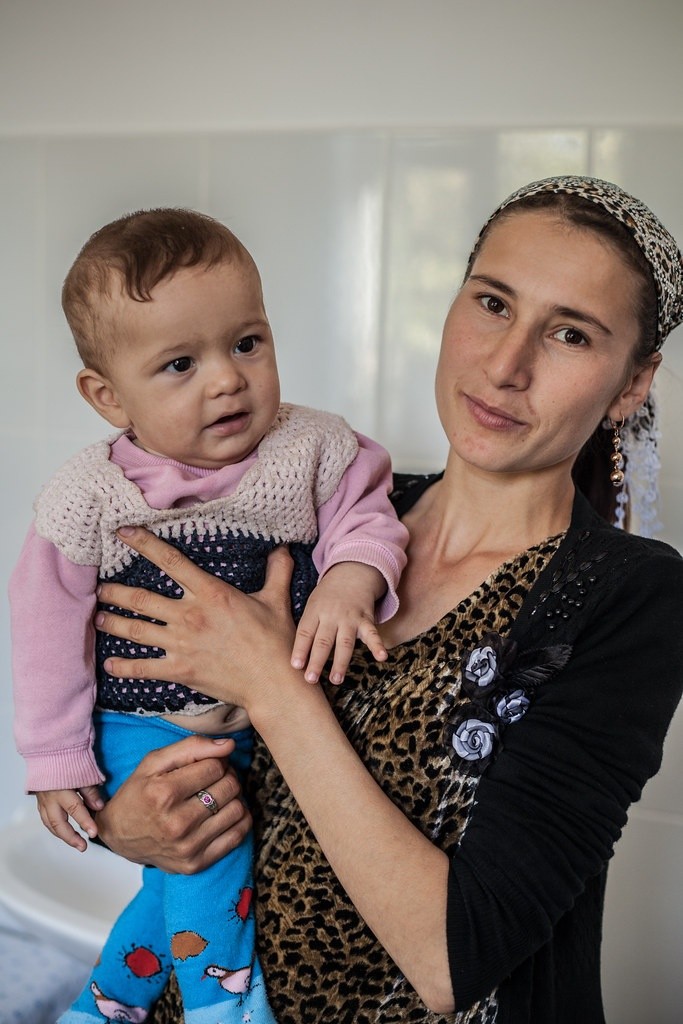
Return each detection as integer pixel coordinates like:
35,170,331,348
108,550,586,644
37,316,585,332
195,790,218,814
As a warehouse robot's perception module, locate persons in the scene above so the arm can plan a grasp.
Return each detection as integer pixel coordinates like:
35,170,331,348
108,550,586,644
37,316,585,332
83,174,681,1023
8,209,411,1022
